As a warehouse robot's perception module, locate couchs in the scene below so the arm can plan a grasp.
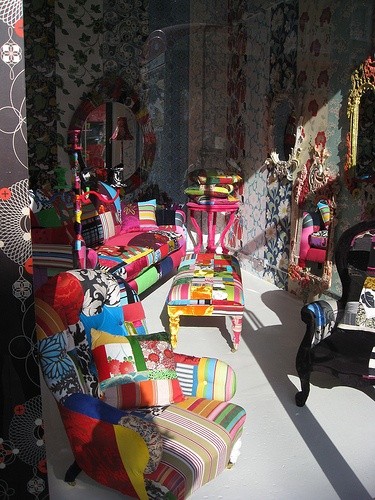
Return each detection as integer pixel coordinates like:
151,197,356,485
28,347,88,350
299,198,336,273
295,220,375,407
74,174,188,297
36,269,247,499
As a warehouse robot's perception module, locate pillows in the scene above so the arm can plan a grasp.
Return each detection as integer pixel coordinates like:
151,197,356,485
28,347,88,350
333,263,375,342
183,184,240,199
317,198,333,231
88,327,189,409
118,197,158,235
193,195,239,204
188,168,243,186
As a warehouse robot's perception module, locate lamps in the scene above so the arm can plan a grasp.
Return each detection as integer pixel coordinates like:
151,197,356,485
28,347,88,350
110,115,134,179
64,127,83,177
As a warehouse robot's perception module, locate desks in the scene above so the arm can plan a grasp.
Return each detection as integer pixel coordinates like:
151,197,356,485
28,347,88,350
185,201,240,253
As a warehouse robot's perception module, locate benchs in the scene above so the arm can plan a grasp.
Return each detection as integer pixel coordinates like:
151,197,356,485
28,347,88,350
167,251,243,352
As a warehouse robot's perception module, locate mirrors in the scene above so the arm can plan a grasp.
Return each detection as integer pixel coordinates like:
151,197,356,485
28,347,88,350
345,56,375,185
265,91,304,175
68,71,157,195
